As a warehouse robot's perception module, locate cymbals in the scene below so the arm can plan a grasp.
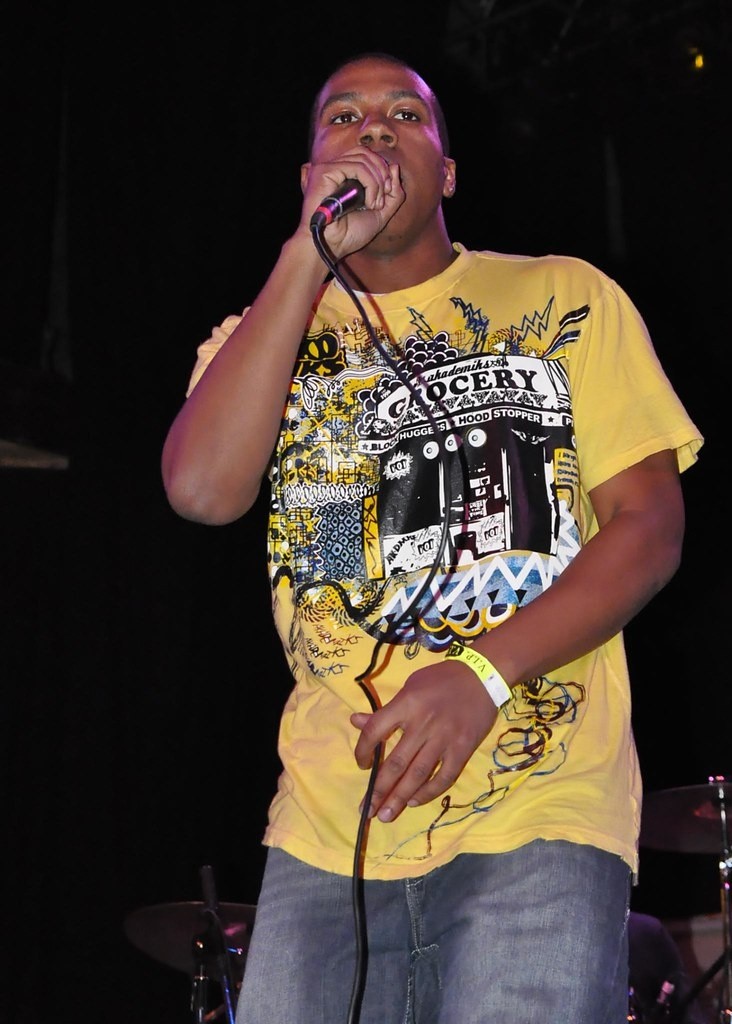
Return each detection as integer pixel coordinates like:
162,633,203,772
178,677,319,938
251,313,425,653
639,785,732,855
123,900,255,981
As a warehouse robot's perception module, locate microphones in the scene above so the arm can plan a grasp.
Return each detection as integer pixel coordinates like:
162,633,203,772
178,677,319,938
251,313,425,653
309,179,366,230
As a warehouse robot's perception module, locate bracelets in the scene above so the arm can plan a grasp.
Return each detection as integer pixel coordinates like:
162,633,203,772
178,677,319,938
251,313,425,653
442,639,513,714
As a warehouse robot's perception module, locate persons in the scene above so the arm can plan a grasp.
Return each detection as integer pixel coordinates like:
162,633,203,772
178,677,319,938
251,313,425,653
623,913,687,1024
157,55,704,1024
